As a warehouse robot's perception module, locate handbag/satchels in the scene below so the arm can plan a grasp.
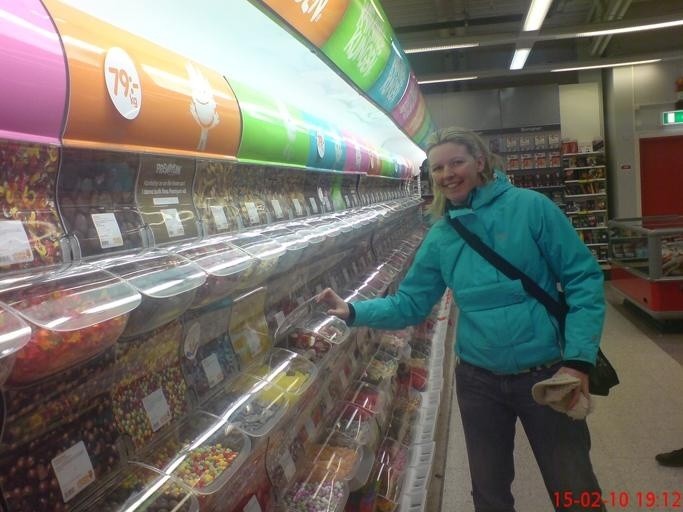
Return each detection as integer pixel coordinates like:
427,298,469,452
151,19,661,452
560,291,619,396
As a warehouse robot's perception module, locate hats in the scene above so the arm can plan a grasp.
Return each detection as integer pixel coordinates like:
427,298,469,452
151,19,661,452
532,374,590,420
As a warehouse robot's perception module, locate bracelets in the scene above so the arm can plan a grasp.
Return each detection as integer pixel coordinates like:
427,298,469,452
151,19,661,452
346,301,356,326
562,360,595,373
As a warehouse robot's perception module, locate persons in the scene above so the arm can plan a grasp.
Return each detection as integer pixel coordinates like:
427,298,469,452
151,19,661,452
314,123,608,512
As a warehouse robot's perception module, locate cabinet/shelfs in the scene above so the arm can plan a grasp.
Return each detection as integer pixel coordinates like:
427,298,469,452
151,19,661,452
418,124,613,277
1,195,454,510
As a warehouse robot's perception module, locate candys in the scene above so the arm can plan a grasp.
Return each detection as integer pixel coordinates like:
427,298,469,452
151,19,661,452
0,138,441,512
